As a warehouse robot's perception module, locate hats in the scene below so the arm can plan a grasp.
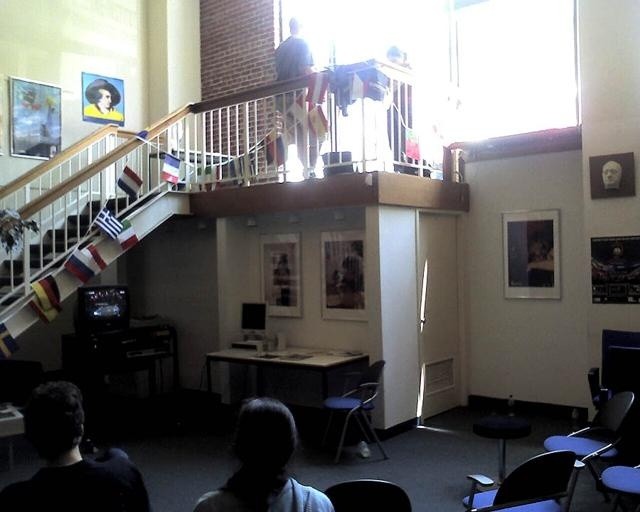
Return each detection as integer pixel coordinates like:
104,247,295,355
85,78,121,106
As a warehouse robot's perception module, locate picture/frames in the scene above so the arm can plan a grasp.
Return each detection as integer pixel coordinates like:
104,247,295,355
589,152,636,200
320,230,368,323
591,235,640,305
9,76,62,162
82,71,125,128
259,231,303,319
501,209,562,300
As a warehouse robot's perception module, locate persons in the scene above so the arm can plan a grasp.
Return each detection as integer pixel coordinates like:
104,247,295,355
336,240,365,308
86,80,121,118
0,381,151,511
524,235,554,288
601,161,623,190
275,16,319,183
195,396,335,512
273,253,291,307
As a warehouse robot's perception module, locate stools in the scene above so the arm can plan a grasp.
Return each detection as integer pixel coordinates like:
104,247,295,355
472,417,531,487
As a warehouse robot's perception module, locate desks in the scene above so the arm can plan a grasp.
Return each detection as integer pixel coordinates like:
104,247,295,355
204,345,366,397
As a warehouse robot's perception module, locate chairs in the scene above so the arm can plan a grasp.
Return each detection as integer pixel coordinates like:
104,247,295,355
601,465,640,512
323,360,390,465
462,449,585,512
543,391,635,512
323,478,412,512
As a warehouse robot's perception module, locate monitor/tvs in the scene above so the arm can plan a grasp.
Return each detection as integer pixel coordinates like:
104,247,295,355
73,284,131,338
238,301,269,333
